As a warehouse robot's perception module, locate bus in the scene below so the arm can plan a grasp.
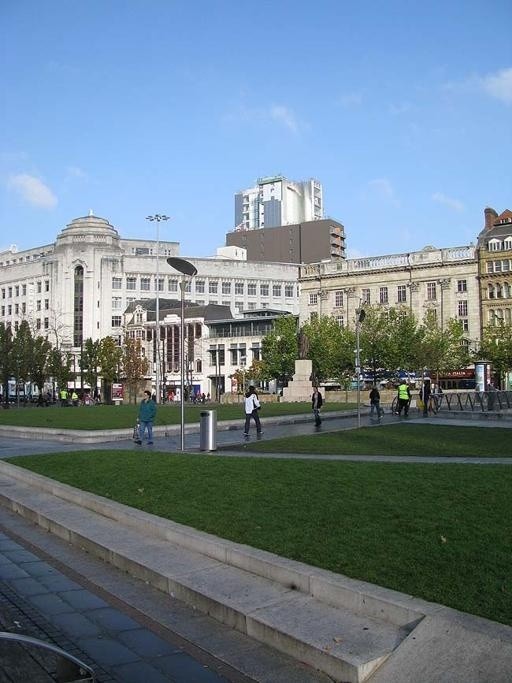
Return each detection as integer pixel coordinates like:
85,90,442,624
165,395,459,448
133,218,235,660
378,377,432,393
317,376,373,391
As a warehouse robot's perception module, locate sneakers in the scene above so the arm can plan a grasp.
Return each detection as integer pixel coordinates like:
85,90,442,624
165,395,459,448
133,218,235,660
134,439,153,445
315,421,321,427
378,415,384,419
244,431,264,435
395,411,399,414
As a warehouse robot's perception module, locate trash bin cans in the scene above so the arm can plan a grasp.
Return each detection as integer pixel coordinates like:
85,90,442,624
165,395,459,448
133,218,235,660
199,410,217,451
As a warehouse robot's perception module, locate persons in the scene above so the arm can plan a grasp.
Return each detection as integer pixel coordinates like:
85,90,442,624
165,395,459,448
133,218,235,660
398,380,412,416
244,386,264,436
370,387,383,418
59,389,79,407
312,386,322,426
133,391,157,444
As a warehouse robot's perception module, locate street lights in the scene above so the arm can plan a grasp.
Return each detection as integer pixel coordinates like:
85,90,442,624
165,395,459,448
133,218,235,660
67,353,77,395
369,339,378,392
353,309,365,434
145,210,174,404
